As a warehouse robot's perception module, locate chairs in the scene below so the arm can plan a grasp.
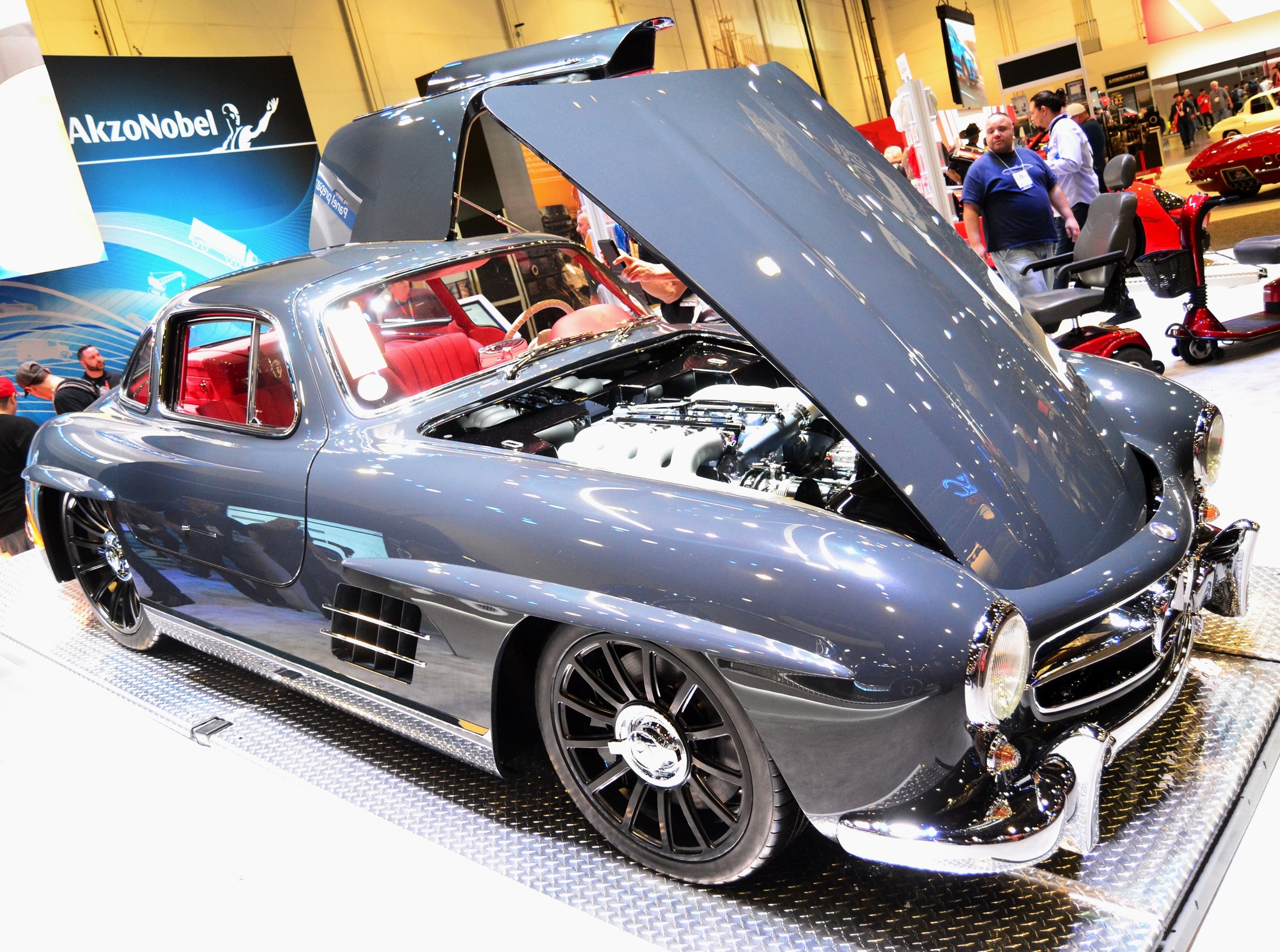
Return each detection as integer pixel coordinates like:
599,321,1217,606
197,385,294,427
1016,153,1138,338
383,331,481,397
1232,235,1280,265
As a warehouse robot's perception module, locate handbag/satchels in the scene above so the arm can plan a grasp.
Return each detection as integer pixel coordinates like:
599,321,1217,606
1275,73,1280,94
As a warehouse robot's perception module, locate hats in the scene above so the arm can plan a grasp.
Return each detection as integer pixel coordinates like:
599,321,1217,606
1147,106,1155,111
1173,93,1181,98
1067,103,1086,118
959,124,983,138
0,376,21,398
14,361,45,398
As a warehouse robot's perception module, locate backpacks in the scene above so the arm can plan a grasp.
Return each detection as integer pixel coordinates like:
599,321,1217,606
1249,79,1258,92
1230,88,1240,103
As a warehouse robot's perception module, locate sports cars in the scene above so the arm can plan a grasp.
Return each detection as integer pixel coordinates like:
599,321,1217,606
951,144,1210,275
1184,87,1280,198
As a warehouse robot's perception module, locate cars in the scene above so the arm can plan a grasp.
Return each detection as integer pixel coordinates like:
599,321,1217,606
22,13,1264,893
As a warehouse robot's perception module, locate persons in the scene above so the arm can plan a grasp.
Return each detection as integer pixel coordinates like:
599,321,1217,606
1091,57,1280,151
0,373,43,558
559,254,591,300
1028,87,1142,328
1064,102,1109,195
946,120,985,184
206,97,279,153
16,361,101,415
378,277,450,322
881,145,910,182
600,230,697,327
574,212,605,307
76,345,123,398
957,111,1082,299
